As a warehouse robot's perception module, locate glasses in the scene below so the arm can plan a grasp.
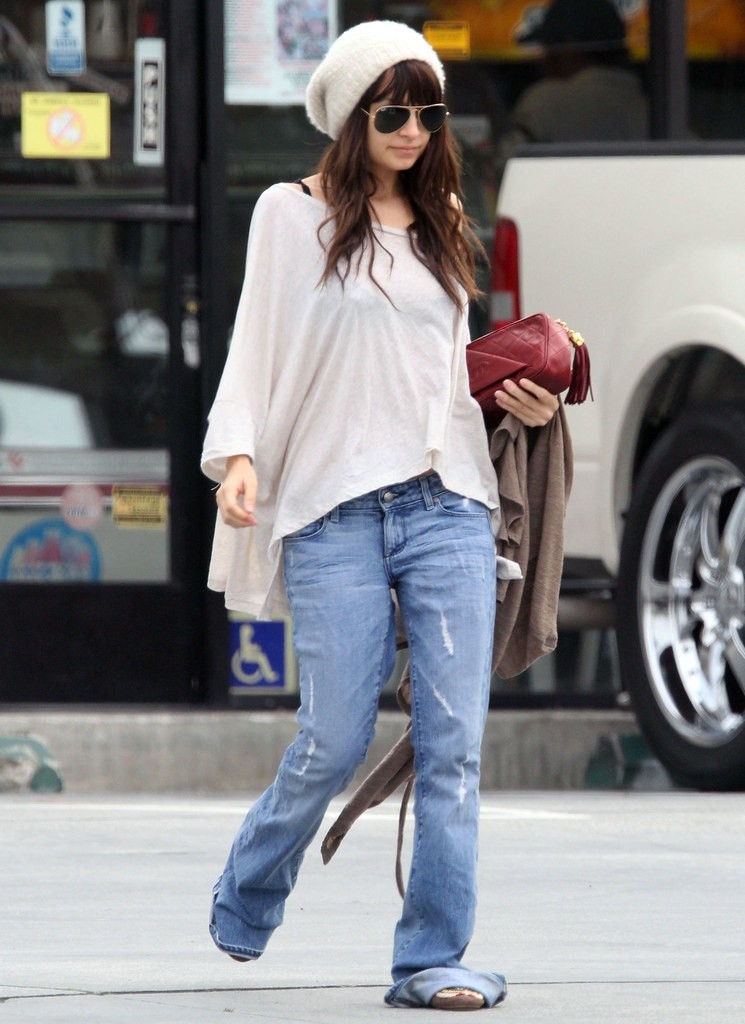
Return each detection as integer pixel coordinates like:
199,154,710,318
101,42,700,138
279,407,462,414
360,104,449,135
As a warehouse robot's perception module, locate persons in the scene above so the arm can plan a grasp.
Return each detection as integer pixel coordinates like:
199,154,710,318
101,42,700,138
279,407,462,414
199,20,562,1011
483,0,650,230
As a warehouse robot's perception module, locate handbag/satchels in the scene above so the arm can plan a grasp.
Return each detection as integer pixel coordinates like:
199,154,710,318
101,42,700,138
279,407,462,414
466,312,594,412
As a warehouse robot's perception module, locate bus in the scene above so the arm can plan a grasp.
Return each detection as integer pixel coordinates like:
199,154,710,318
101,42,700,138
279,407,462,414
1,0,743,791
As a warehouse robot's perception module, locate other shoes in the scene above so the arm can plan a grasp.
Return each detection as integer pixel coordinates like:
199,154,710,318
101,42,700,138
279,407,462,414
429,987,485,1010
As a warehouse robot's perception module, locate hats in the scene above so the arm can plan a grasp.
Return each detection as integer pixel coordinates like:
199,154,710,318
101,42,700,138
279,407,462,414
305,20,445,141
